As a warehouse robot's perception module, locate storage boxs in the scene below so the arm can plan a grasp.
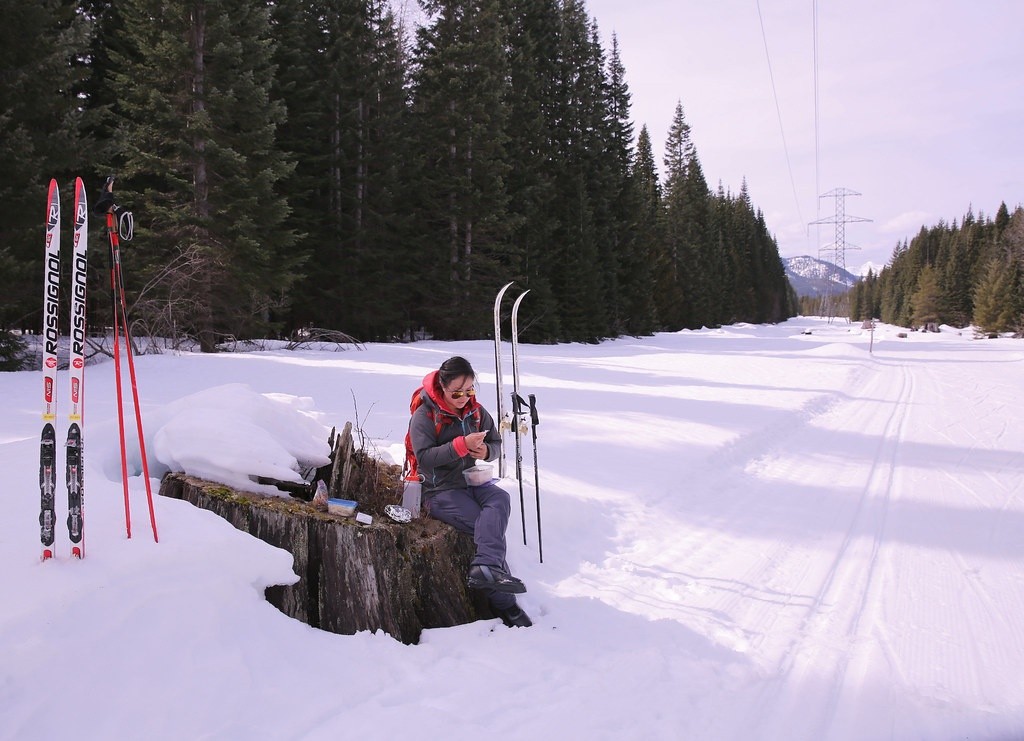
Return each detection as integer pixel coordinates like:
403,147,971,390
462,465,495,487
327,497,358,517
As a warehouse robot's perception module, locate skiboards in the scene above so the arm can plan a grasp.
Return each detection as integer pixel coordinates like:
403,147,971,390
37,176,92,562
492,280,532,478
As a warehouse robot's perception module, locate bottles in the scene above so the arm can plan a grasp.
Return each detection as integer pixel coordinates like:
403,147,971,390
401,468,425,519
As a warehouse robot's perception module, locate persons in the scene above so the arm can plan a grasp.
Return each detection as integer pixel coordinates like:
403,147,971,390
406,357,532,628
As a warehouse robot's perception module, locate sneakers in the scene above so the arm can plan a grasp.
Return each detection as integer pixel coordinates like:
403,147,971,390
498,604,533,628
469,564,527,593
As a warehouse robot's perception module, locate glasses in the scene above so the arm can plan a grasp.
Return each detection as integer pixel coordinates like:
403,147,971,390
444,384,475,399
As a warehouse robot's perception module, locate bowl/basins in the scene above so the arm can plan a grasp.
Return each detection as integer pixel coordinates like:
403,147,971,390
462,465,494,486
356,512,373,525
328,497,358,517
